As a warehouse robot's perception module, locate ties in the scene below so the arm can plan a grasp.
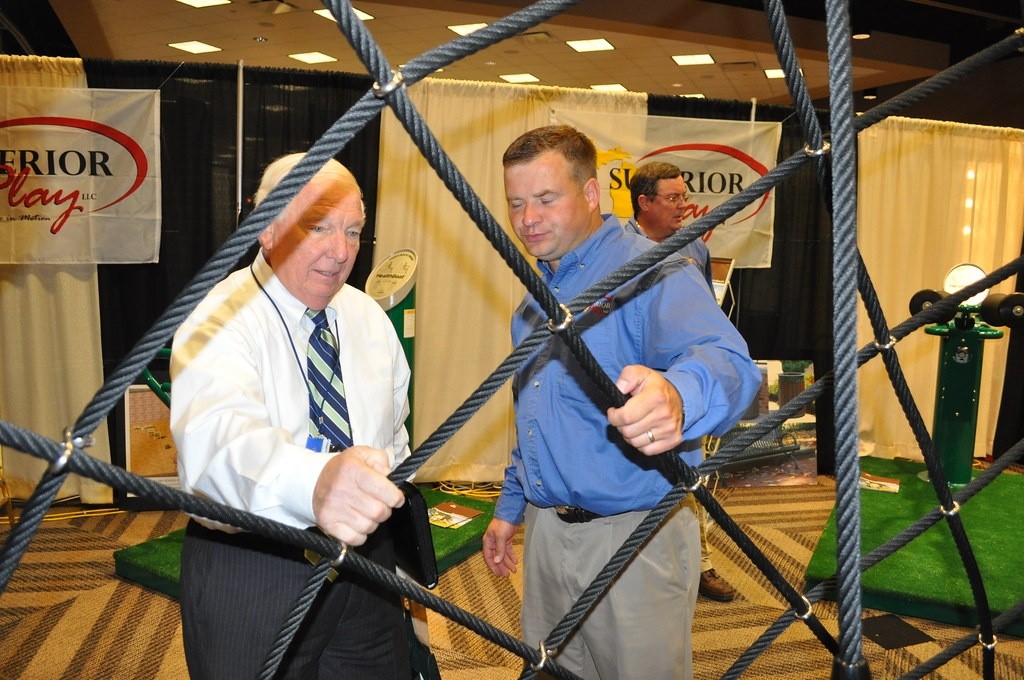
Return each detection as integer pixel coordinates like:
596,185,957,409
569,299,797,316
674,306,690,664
303,307,355,454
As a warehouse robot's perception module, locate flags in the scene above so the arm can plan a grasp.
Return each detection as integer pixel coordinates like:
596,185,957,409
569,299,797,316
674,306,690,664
1,88,161,265
645,116,782,269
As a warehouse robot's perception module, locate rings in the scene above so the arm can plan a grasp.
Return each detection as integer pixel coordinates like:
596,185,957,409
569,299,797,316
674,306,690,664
647,431,656,444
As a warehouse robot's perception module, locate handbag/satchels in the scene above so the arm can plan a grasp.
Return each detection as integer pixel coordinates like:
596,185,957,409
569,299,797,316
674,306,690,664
396,482,439,590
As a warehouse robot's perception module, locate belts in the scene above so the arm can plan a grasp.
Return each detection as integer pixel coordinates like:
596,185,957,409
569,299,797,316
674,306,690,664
550,506,600,523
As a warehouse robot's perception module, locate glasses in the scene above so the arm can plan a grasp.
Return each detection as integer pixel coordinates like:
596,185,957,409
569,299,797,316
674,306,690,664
653,192,693,205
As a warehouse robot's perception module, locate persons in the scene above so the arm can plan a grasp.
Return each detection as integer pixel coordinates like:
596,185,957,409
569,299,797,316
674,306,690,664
620,159,742,602
482,127,763,679
171,153,439,680
